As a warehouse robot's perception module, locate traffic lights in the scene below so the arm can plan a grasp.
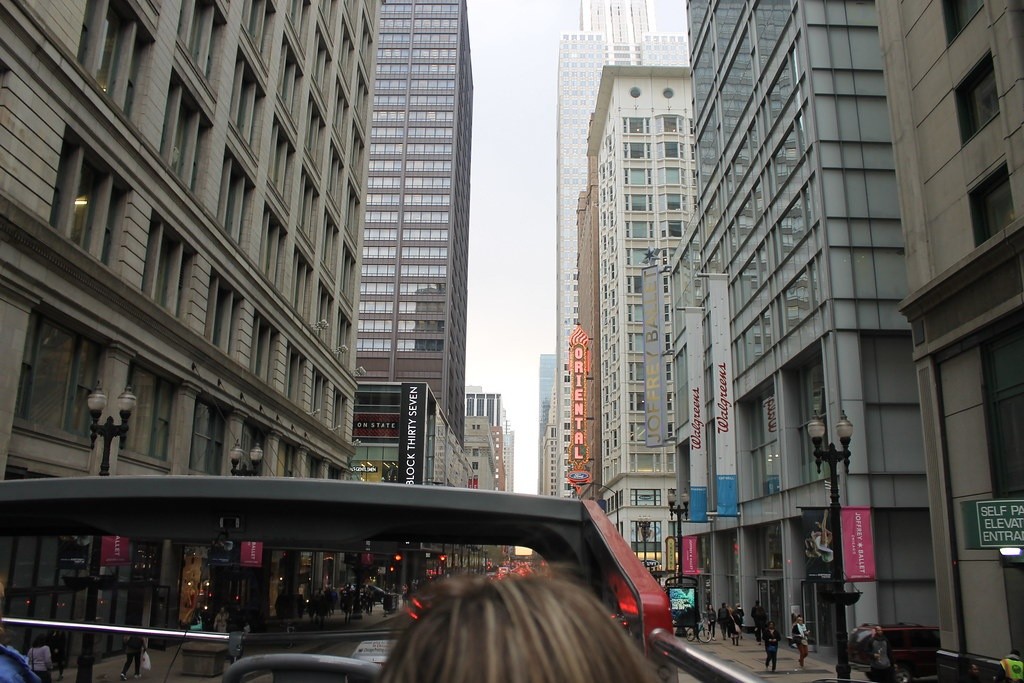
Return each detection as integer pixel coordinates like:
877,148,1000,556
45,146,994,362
441,556,445,568
395,553,402,564
390,566,396,572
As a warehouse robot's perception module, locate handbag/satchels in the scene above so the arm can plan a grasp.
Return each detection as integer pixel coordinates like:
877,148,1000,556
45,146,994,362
191,623,202,630
141,651,151,671
767,642,777,651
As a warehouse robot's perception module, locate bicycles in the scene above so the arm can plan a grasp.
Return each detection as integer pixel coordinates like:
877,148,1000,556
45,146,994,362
686,618,712,643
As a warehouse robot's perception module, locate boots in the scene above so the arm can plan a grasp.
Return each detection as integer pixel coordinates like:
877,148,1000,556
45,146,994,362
731,637,735,645
736,637,738,646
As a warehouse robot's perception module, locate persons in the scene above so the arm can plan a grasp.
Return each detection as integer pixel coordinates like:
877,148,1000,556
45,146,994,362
866,626,899,683
707,599,810,672
806,509,833,574
0,574,666,683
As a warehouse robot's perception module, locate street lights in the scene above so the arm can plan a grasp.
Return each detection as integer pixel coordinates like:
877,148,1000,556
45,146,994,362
667,489,690,637
222,440,263,633
75,380,136,683
807,408,858,683
576,482,619,532
638,513,651,569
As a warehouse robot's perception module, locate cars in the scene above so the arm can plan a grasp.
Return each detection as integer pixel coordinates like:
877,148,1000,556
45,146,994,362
341,584,386,604
485,572,498,580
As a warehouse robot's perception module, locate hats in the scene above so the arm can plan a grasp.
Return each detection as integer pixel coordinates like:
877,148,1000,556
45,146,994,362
736,603,741,609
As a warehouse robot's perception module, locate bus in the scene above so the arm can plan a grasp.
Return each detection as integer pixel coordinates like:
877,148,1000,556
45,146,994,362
498,567,508,575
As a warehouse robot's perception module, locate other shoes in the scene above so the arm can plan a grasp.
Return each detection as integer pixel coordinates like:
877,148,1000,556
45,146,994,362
133,673,141,678
712,637,715,641
120,673,128,681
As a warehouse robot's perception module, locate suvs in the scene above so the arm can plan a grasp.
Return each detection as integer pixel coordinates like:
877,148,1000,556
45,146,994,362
847,622,940,681
